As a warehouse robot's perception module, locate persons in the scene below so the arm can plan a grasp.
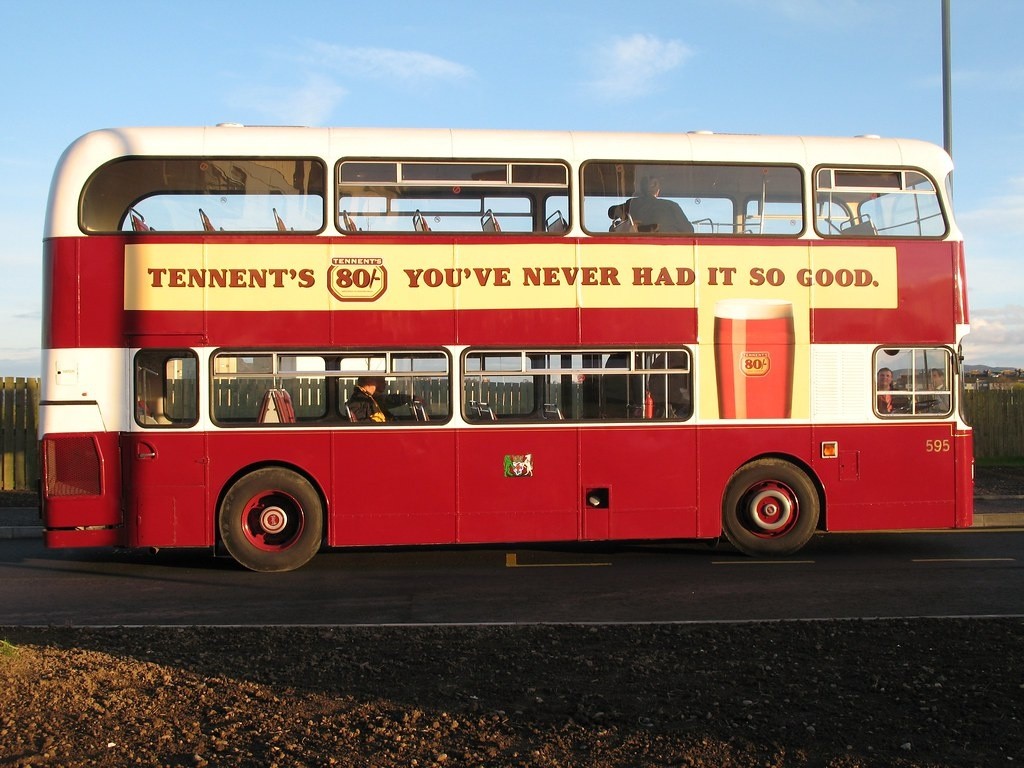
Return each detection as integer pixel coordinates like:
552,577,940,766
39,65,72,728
609,177,694,233
349,376,424,423
877,368,907,413
931,368,945,403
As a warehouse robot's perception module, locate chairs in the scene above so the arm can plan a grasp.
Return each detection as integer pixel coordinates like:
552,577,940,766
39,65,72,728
840,214,879,235
480,210,502,232
543,211,571,232
344,209,366,231
468,401,499,421
257,389,296,423
272,208,295,232
200,209,228,230
344,401,360,424
129,207,157,231
413,210,432,231
135,400,159,424
612,212,638,232
543,403,566,419
411,400,431,421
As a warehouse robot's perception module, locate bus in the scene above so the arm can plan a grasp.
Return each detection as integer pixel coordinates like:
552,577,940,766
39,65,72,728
39,121,975,576
